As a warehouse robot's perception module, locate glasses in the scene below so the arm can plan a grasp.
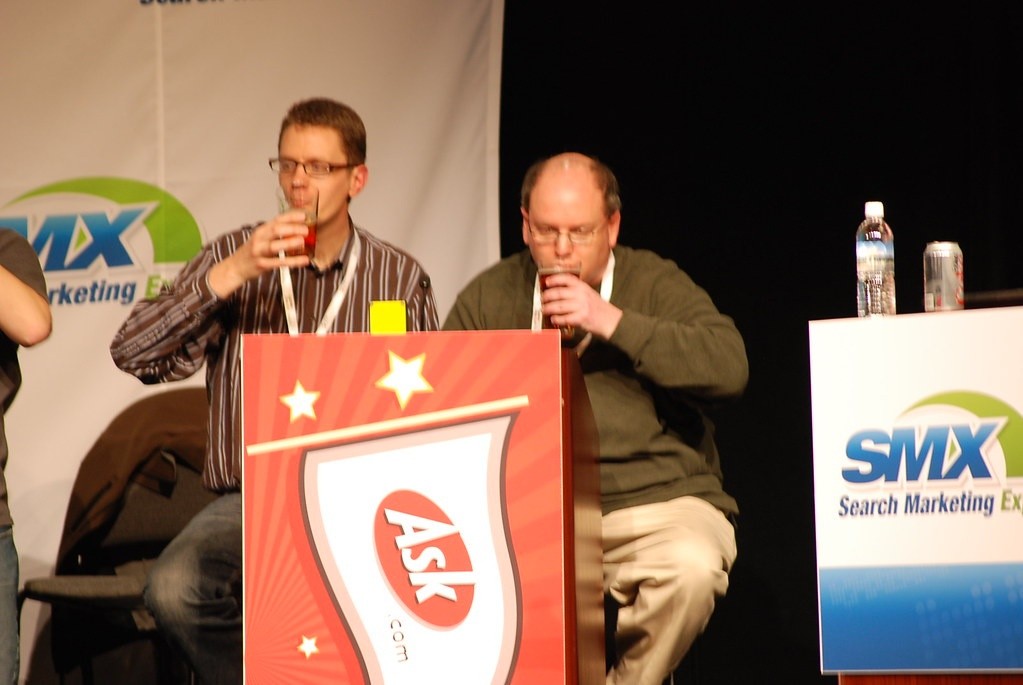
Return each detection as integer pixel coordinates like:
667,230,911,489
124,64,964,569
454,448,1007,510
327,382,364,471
269,157,353,175
526,217,610,246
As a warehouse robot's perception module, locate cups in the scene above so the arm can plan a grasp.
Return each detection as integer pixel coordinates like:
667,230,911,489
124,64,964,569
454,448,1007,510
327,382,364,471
274,180,321,260
538,254,582,341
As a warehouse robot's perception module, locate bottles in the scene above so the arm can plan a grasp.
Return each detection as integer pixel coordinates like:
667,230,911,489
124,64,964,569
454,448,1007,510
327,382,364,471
855,199,896,318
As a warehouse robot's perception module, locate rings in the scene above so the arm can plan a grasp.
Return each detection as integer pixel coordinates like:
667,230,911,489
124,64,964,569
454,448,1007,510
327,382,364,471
269,241,272,255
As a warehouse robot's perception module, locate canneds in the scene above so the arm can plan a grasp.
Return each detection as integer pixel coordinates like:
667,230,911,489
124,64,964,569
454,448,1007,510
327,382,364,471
924,241,965,313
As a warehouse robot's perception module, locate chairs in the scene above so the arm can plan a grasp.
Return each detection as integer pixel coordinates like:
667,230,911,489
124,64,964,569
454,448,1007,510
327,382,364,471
49,387,218,685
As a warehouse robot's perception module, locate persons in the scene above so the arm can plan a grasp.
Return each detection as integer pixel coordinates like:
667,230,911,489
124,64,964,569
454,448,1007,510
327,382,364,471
443,152,749,685
0,226,53,685
109,98,442,685
